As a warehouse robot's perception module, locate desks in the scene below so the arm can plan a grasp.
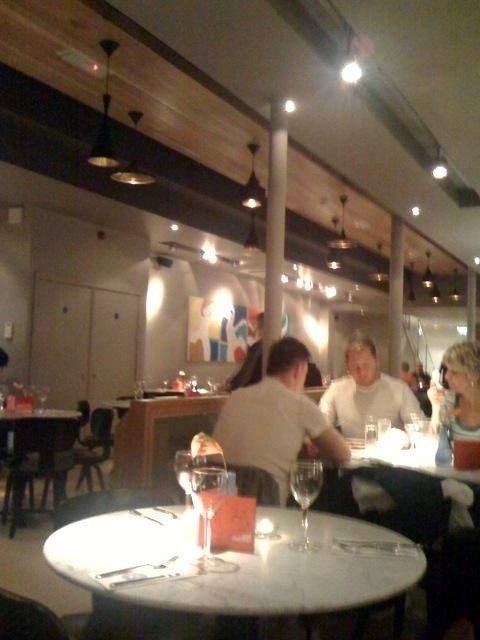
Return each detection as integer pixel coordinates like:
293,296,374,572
0,407,73,523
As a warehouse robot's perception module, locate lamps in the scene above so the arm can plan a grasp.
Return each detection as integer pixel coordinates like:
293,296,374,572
110,108,155,186
241,135,270,208
90,40,122,169
311,180,471,303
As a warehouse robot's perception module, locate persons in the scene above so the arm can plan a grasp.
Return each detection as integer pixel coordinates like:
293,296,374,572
312,333,426,440
425,340,479,445
223,310,265,390
0,348,41,531
303,361,324,387
411,362,432,391
210,334,351,510
399,361,418,397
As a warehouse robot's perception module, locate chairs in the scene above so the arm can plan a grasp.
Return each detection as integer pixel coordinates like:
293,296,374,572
327,465,462,638
1,577,63,638
7,426,71,535
74,401,90,418
206,461,279,507
76,409,110,493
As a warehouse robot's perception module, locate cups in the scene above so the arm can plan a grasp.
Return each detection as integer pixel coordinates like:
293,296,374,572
359,410,437,456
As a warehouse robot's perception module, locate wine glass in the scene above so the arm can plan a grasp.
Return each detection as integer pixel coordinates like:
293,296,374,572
286,455,325,554
22,384,50,415
191,375,198,392
173,449,207,526
207,374,216,392
189,467,229,569
